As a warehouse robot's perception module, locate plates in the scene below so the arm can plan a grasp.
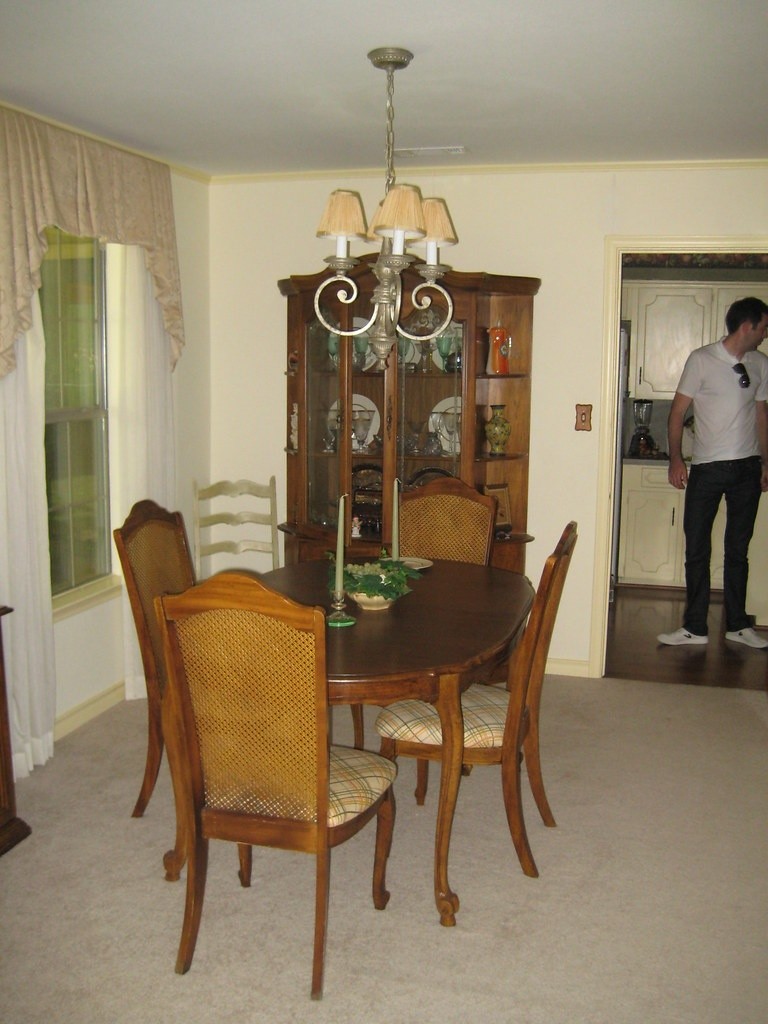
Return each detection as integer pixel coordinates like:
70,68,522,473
327,393,380,450
328,317,380,370
431,323,463,370
379,556,433,570
396,331,423,366
428,396,462,452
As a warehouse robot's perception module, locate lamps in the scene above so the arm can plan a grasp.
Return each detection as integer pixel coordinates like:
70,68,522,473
314,46,458,370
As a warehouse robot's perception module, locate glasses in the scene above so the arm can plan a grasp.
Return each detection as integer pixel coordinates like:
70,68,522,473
733,362,751,389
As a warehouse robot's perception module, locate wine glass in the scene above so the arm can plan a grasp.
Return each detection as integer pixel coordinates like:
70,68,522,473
327,336,339,369
316,409,340,453
405,415,428,454
351,410,376,453
354,337,369,369
430,412,446,454
442,413,459,456
436,337,453,374
397,337,411,364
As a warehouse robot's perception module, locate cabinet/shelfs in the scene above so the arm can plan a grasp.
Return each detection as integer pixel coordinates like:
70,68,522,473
278,251,540,576
636,279,768,401
620,457,725,589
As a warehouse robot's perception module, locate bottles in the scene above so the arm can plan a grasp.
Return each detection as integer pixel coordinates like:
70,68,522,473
308,306,339,372
485,327,511,374
483,404,512,456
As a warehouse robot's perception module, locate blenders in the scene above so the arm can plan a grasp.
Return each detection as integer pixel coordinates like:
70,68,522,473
628,399,655,458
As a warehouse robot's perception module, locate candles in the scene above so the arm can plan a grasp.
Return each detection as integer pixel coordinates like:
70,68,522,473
393,478,400,562
334,493,348,605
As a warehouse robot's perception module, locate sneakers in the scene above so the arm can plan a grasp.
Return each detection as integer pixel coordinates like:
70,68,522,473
656,628,708,645
725,627,768,647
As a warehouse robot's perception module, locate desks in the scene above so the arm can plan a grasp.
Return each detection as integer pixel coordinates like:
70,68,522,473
169,555,535,911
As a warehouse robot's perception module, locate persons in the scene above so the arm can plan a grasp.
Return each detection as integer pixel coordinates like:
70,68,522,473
655,296,768,649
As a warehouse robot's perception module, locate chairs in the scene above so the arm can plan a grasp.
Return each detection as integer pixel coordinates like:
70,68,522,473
371,522,580,877
195,476,281,580
151,572,397,1002
400,480,500,773
113,499,212,816
